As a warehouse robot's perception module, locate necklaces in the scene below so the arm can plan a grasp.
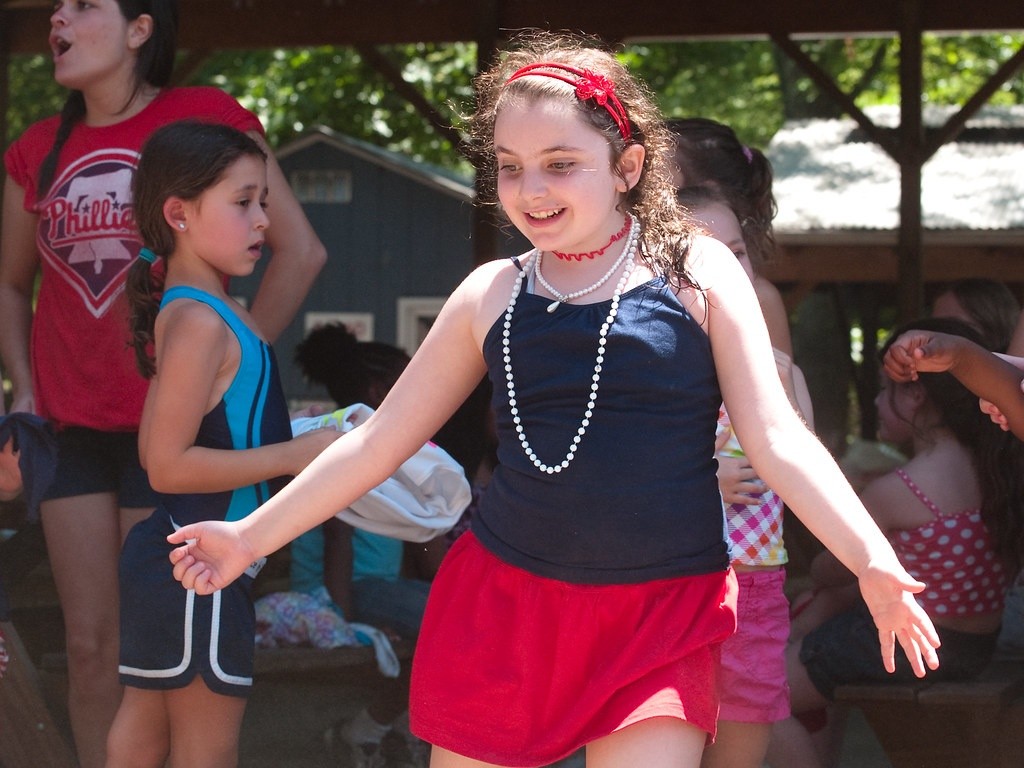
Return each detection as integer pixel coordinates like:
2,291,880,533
547,213,631,262
533,208,632,311
503,211,643,474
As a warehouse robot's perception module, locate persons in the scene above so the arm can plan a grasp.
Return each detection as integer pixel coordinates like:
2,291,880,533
103,117,350,766
160,43,944,768
0,0,326,766
763,261,1024,768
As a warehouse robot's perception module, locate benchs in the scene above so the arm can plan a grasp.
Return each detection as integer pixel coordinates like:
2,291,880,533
237,635,427,768
831,648,1024,768
